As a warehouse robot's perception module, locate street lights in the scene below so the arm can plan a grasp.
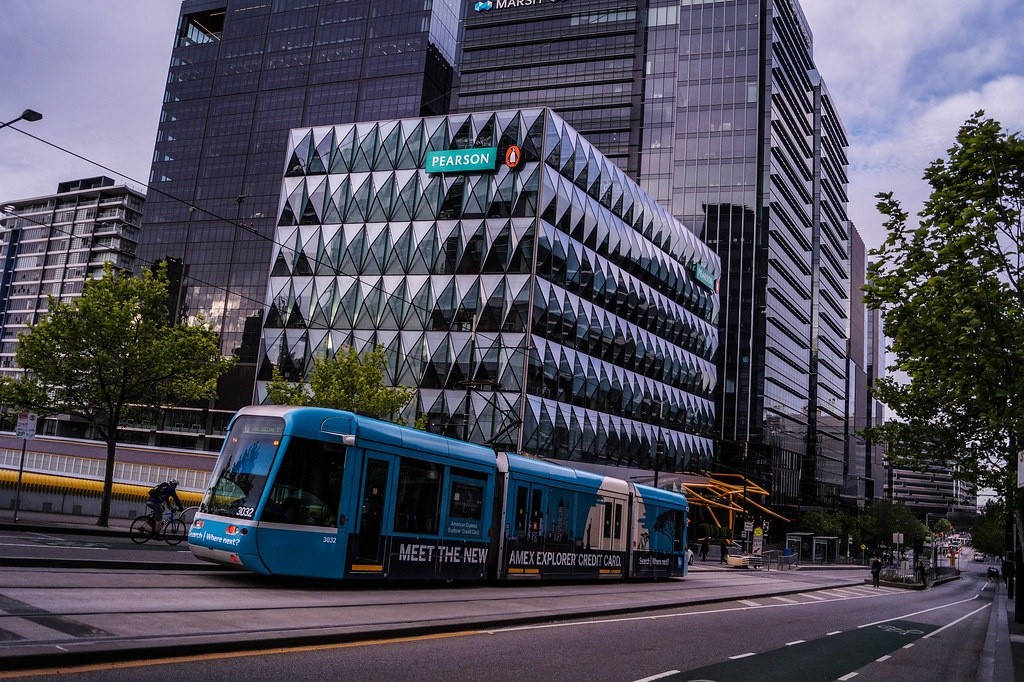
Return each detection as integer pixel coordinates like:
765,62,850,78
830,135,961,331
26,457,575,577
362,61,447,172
431,345,539,442
629,476,657,487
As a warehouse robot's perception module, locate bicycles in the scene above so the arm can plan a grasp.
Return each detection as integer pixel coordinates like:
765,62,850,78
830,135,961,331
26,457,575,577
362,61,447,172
129,504,187,545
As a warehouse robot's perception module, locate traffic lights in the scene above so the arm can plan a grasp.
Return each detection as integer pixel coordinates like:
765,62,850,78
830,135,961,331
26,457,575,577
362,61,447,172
849,538,853,544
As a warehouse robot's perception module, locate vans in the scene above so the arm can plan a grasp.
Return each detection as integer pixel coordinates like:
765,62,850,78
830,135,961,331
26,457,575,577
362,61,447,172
893,551,908,560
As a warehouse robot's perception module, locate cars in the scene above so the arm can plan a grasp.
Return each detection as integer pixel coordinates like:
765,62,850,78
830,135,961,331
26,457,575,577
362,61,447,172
987,568,999,577
974,553,984,561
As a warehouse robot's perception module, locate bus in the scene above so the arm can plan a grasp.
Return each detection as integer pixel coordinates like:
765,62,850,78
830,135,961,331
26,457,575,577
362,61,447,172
948,542,962,558
188,405,689,584
951,537,972,546
923,532,935,548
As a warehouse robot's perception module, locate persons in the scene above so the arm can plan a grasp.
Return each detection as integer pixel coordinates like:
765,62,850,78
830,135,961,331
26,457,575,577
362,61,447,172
719,541,728,565
147,478,185,541
871,557,882,588
700,538,709,562
987,566,994,583
915,561,927,589
